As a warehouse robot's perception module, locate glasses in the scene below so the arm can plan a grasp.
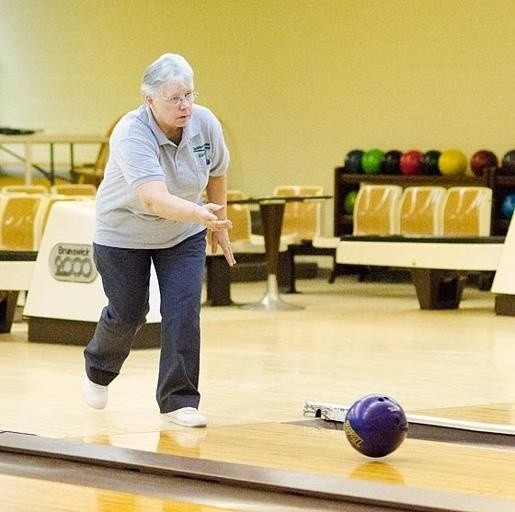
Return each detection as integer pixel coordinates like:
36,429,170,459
154,90,200,106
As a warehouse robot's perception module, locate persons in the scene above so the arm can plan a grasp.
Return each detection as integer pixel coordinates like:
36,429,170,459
83,52,236,427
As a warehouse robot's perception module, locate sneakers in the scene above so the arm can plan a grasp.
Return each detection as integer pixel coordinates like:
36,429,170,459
162,405,210,428
80,356,108,410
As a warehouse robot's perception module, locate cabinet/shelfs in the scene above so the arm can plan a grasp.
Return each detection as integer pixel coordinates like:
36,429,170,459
332,166,515,291
